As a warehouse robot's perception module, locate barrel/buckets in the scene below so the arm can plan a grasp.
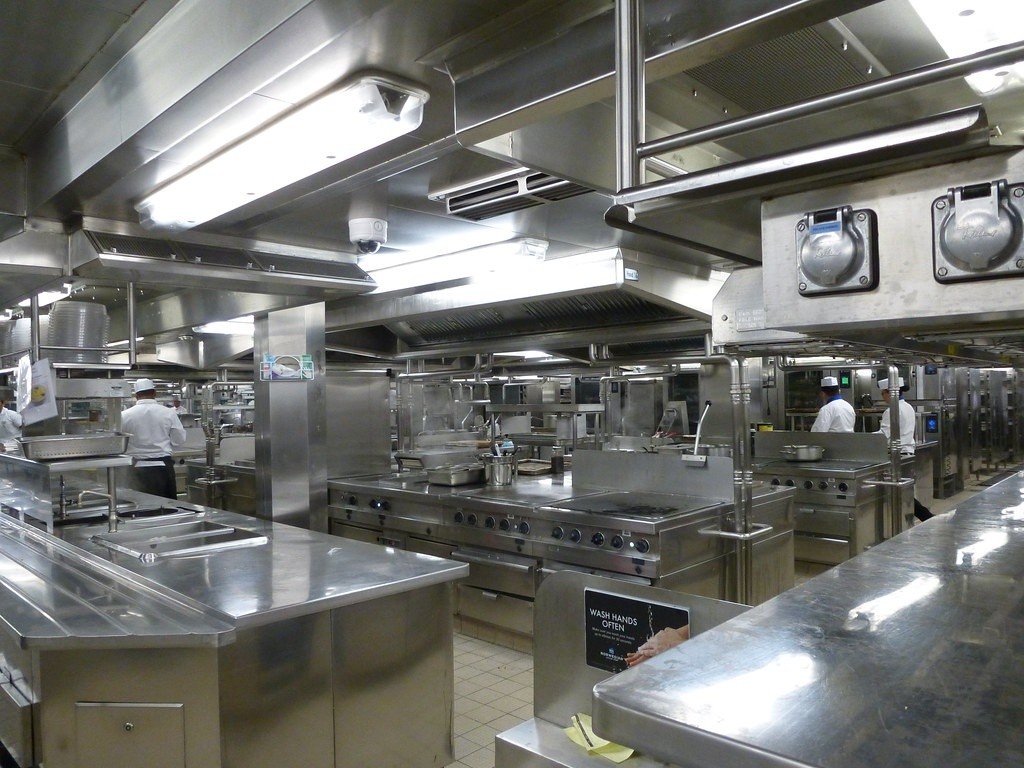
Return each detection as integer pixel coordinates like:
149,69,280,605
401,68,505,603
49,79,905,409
481,451,514,487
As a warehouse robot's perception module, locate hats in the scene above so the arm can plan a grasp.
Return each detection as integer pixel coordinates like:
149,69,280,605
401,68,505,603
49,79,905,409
134,378,154,394
877,377,904,390
821,376,838,387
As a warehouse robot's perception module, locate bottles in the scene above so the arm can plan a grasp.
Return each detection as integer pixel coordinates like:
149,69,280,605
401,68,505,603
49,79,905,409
549,446,565,474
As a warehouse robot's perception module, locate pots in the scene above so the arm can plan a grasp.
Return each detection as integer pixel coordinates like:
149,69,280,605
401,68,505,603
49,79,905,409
654,443,733,458
781,441,829,463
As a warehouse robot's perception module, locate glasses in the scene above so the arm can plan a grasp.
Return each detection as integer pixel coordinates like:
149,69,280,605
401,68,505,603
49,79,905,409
882,391,889,396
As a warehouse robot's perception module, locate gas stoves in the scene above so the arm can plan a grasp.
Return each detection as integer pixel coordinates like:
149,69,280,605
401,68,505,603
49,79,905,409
445,475,721,577
751,455,886,508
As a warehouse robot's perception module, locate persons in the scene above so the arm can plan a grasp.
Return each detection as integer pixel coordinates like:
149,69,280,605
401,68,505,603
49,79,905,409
812,377,856,433
169,398,188,414
120,378,187,500
624,625,689,666
871,377,934,523
0,398,22,452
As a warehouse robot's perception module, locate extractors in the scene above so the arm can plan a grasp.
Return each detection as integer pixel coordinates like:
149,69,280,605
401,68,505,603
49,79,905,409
382,288,705,347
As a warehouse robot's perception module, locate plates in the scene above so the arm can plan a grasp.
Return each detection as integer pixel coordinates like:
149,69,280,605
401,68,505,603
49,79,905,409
9,315,49,365
45,301,112,364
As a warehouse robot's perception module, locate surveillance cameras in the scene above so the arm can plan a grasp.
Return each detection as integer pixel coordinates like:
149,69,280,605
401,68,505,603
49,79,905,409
348,218,388,254
